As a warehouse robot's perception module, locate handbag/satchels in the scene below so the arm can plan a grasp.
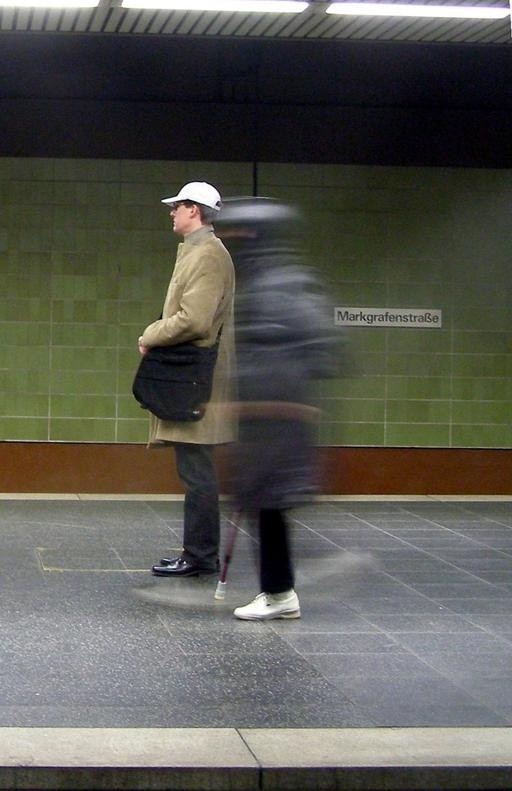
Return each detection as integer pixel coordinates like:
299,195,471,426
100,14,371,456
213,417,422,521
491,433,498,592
133,343,218,421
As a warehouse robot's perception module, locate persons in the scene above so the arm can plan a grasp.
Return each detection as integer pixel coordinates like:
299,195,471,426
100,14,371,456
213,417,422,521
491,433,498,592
138,181,237,579
210,196,350,621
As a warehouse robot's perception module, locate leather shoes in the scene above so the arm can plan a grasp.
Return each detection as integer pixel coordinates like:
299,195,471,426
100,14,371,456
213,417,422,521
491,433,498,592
151,556,220,578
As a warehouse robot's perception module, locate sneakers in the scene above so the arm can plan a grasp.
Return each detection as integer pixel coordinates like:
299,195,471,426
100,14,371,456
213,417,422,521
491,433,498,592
234,590,301,620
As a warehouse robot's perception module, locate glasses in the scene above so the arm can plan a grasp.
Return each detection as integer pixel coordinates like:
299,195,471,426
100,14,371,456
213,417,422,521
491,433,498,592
175,203,188,211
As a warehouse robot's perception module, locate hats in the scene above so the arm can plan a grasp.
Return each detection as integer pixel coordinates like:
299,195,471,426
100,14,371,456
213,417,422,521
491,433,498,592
161,182,222,212
